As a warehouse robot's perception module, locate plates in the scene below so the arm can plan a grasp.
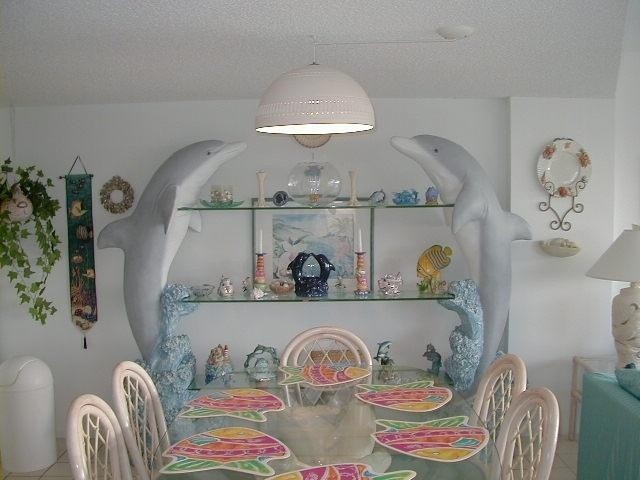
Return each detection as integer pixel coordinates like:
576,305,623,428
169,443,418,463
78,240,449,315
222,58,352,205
199,199,244,208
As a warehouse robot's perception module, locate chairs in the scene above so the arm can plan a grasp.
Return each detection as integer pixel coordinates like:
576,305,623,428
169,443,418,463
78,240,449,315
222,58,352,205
284,371,373,408
492,387,560,479
110,361,171,479
64,394,132,480
466,354,527,480
280,324,373,375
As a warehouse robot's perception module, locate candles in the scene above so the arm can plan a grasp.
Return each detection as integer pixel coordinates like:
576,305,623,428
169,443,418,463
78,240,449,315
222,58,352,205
256,226,263,254
357,229,362,252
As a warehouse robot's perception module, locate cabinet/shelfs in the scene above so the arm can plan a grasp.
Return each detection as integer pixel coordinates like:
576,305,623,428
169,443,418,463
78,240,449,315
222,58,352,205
173,199,457,390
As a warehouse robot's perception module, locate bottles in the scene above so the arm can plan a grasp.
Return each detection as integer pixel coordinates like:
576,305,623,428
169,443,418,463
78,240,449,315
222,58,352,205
211,185,233,204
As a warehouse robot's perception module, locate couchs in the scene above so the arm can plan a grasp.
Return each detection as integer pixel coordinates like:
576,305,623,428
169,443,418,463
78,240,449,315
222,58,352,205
577,369,640,480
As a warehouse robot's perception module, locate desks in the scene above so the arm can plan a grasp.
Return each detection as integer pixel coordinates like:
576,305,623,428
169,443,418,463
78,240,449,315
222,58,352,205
148,364,502,479
568,355,616,440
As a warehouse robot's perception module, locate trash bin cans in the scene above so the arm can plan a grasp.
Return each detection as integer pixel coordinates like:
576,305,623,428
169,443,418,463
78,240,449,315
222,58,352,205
0,356,57,474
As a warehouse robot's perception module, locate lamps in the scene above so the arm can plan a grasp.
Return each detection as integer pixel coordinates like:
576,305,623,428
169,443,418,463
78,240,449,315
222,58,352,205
583,228,639,369
254,35,376,135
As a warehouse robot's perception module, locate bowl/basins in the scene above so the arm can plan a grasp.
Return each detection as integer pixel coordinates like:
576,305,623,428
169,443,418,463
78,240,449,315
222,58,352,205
189,285,215,299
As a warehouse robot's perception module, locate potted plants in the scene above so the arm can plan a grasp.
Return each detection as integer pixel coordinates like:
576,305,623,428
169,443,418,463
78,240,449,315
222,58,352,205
0,155,62,327
